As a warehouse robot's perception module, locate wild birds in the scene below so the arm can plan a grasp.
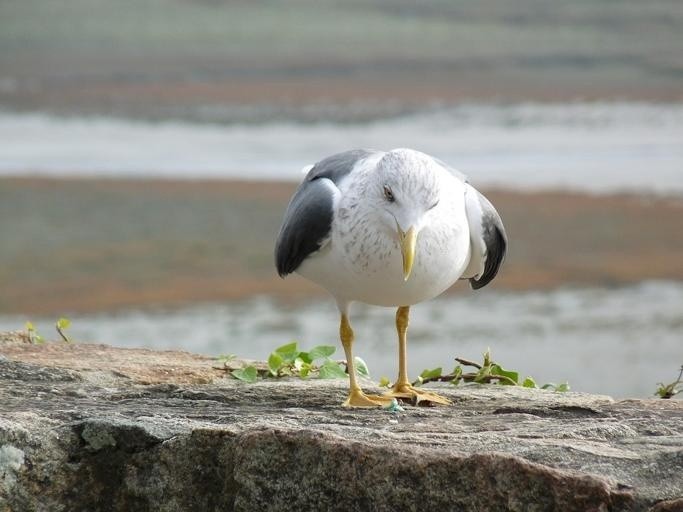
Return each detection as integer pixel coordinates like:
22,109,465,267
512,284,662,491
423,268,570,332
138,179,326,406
273,149,509,408
